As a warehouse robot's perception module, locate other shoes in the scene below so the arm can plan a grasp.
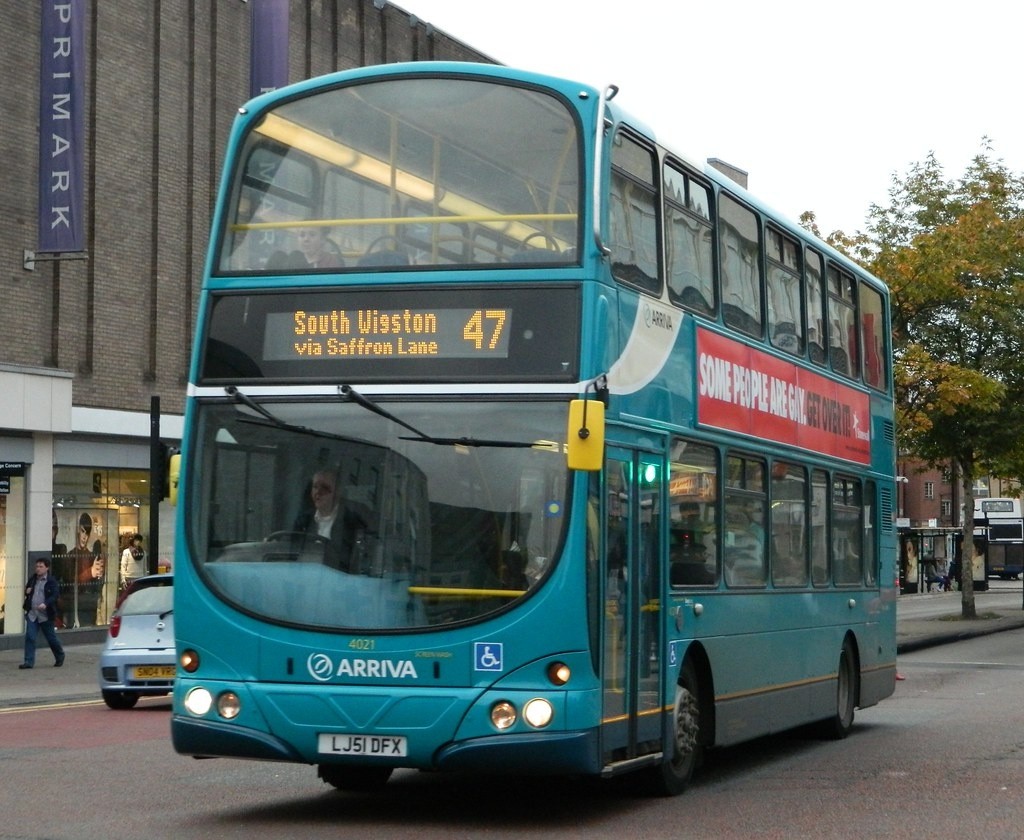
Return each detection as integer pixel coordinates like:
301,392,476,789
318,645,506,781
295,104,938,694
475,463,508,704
19,664,33,669
54,652,65,667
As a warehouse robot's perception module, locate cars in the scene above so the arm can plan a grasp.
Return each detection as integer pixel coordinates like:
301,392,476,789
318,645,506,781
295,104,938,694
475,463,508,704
96,574,176,709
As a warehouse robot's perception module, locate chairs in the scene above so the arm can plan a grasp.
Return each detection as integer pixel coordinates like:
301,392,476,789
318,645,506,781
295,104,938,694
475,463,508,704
323,233,576,263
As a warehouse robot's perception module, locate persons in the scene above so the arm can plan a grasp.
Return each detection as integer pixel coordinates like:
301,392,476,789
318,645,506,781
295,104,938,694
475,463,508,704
52,509,105,630
287,468,356,575
924,550,955,592
18,558,65,669
906,539,917,582
120,534,147,592
245,218,342,271
961,540,985,580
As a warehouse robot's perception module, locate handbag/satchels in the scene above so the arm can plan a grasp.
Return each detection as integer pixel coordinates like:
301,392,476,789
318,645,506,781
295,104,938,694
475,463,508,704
22,573,36,611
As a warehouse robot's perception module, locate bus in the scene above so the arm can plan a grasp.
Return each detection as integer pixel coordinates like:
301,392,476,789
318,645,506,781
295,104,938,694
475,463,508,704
170,62,904,800
960,497,1024,579
